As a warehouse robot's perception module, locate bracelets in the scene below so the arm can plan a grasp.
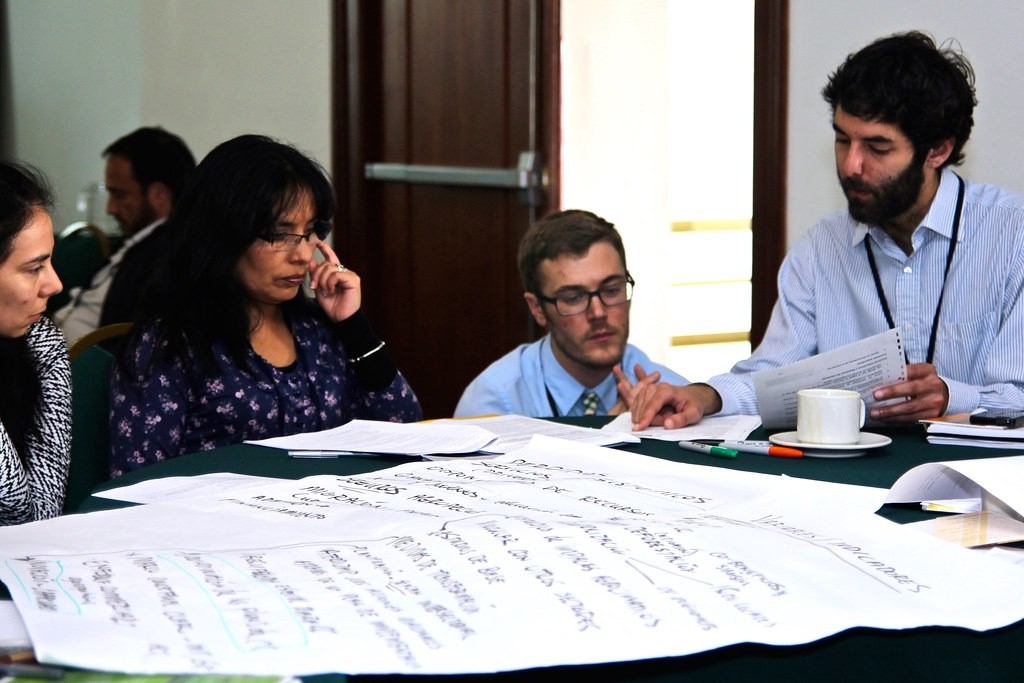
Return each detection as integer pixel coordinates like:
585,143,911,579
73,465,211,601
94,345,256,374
349,341,385,366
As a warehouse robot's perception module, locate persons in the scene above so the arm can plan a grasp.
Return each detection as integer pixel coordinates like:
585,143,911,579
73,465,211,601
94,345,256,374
0,126,422,526
630,33,1024,431
452,210,691,418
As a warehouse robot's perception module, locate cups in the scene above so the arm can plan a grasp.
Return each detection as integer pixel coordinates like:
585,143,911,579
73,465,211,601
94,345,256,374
797,388,865,445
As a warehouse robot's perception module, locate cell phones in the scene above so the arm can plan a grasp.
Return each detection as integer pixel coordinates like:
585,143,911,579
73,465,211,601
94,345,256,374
970,409,1024,429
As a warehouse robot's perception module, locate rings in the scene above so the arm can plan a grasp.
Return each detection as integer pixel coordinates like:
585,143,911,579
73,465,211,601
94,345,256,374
335,263,343,272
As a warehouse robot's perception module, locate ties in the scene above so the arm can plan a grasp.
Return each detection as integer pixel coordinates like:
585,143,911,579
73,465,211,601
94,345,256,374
580,393,599,416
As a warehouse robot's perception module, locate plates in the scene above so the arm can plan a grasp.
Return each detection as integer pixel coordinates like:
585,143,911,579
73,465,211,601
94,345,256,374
769,430,892,458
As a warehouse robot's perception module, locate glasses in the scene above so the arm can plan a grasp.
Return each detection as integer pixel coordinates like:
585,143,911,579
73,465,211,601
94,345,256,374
259,227,327,252
537,272,635,317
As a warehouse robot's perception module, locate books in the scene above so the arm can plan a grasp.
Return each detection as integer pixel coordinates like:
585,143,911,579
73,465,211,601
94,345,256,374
918,408,1024,449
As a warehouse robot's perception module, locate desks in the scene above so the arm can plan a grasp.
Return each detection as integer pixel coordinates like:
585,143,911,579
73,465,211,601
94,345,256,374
69,414,1024,683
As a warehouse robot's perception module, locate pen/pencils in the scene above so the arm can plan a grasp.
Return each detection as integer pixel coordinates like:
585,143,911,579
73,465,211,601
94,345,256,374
0,662,67,681
0,649,35,664
719,442,803,459
678,441,739,459
